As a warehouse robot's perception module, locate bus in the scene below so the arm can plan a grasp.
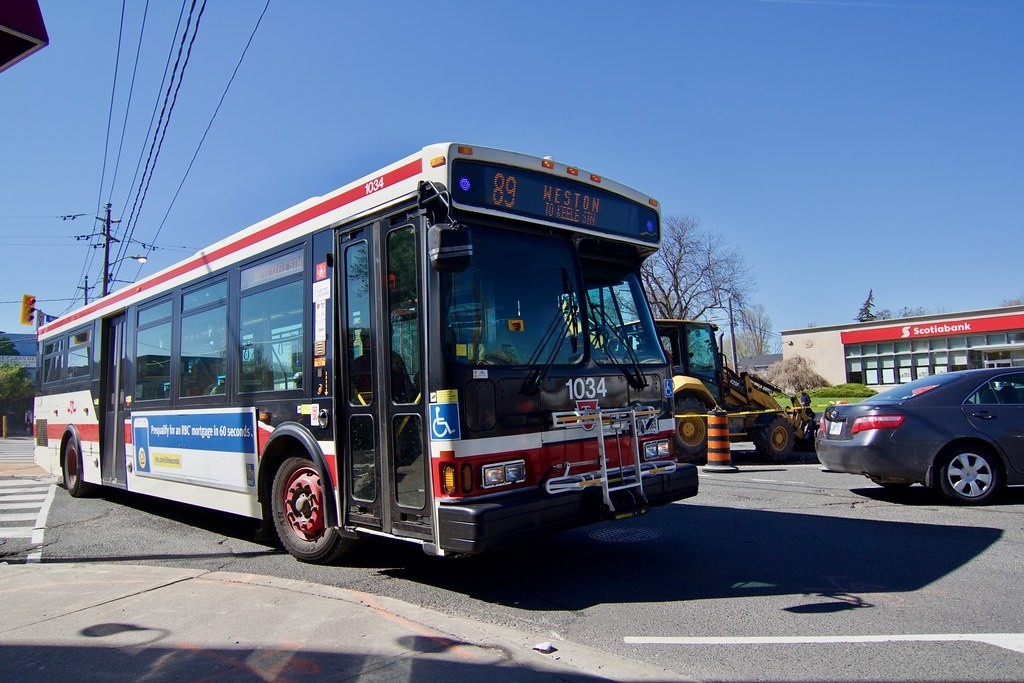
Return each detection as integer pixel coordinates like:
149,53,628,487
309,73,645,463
32,145,699,565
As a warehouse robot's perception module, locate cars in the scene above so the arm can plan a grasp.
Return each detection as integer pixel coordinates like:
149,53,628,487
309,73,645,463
820,367,1024,502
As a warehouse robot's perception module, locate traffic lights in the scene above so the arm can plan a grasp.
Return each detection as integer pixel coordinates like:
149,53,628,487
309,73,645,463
20,295,36,325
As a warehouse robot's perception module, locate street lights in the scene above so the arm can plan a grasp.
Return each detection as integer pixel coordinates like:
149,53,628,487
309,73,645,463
101,255,147,295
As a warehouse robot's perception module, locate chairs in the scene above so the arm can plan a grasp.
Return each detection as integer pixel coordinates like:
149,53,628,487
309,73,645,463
981,385,1024,403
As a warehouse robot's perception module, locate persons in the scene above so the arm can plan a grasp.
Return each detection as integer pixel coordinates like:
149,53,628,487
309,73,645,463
7,405,16,431
354,322,423,468
799,388,811,416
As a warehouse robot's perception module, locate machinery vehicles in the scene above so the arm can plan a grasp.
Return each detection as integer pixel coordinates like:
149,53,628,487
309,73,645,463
563,300,821,460
